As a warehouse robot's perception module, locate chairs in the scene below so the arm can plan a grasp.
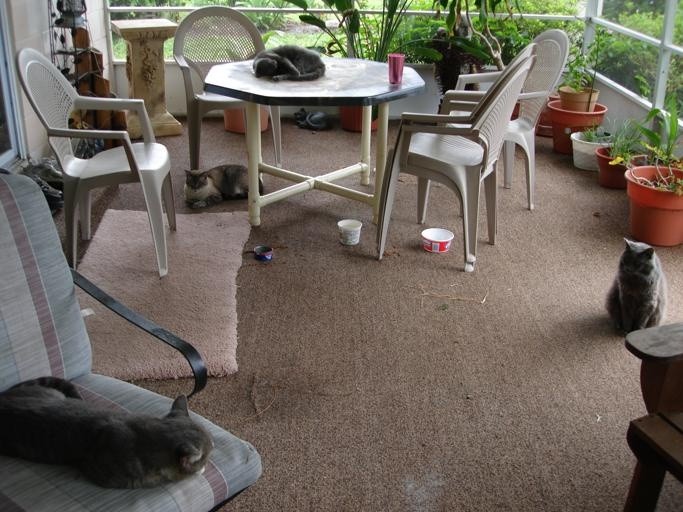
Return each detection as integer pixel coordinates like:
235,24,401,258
14,45,179,279
434,26,568,213
168,7,285,177
1,173,262,512
371,41,536,275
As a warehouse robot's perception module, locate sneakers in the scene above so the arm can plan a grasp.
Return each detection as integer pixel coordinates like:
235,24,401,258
18,172,64,202
23,164,64,183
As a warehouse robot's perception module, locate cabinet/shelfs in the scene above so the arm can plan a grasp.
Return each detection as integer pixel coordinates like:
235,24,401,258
45,1,129,151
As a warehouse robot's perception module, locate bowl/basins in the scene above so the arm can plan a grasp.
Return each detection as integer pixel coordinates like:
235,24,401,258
419,225,456,254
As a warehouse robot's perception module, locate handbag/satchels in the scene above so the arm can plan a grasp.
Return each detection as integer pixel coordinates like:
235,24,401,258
75,121,105,159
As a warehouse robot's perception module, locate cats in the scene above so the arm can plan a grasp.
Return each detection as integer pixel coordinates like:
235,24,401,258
0,375,215,491
253,46,325,82
183,164,264,209
605,237,667,336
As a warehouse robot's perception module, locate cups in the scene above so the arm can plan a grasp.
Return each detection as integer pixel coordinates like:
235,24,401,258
336,218,362,248
388,51,405,87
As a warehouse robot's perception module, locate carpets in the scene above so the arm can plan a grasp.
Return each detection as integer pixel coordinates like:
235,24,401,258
74,209,252,380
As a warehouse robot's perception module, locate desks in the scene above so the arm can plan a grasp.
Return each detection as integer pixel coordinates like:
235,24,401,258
199,54,429,230
108,17,186,141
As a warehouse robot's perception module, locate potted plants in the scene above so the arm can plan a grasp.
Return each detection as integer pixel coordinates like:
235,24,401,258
285,0,413,133
548,24,682,248
